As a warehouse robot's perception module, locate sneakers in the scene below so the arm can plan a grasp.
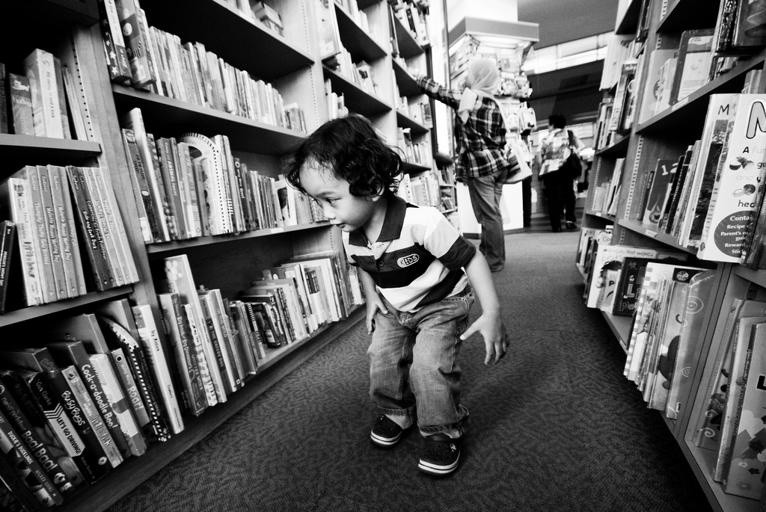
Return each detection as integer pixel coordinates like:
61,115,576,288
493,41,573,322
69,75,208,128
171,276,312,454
370,414,403,448
416,432,462,476
565,222,577,229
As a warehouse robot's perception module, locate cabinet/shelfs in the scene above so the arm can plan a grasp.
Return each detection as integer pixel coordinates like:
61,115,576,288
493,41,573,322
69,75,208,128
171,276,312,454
574,2,764,511
447,31,537,138
0,0,461,511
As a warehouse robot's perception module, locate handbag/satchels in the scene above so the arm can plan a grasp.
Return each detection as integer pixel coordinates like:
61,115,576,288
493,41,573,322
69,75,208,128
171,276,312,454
503,133,533,184
566,153,581,180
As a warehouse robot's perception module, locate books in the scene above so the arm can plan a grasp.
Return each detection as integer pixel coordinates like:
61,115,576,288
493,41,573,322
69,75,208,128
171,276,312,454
0,2,435,512
571,1,765,500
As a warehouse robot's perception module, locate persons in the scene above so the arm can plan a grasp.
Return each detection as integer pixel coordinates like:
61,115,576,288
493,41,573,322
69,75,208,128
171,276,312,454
414,55,512,272
282,112,510,475
537,112,595,232
